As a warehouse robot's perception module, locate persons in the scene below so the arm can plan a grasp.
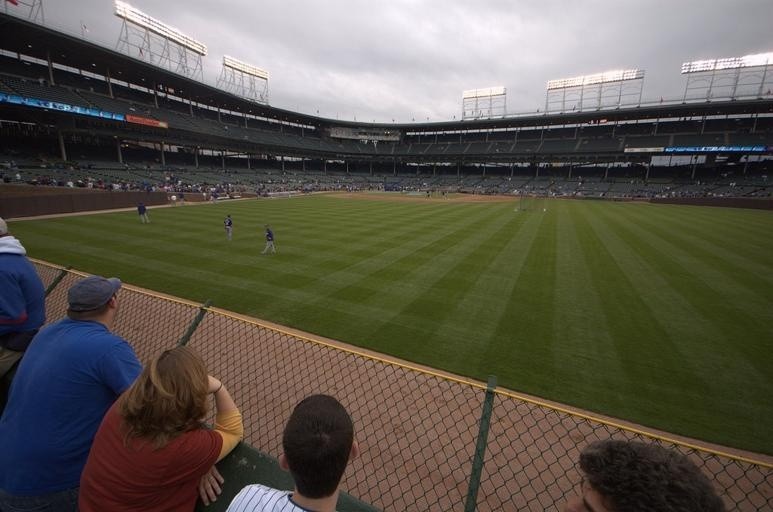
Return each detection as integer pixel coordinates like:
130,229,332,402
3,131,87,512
1,153,189,203
226,393,358,512
0,217,47,377
259,223,276,257
385,152,768,206
223,214,233,242
0,276,145,512
137,201,151,224
560,439,726,512
189,153,385,205
76,346,243,511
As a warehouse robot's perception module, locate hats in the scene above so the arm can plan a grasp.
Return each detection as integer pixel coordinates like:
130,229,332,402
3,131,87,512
67,276,121,312
0,217,8,236
